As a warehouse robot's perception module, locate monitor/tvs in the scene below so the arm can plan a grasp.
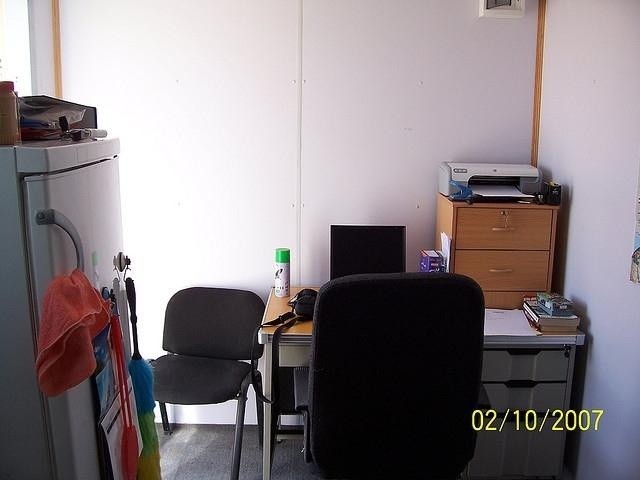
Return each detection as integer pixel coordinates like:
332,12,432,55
329,225,406,281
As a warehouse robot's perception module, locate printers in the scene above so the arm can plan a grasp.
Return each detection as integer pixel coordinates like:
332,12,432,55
437,161,543,197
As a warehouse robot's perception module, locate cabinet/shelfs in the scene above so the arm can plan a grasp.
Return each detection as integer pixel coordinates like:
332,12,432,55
436,190,560,309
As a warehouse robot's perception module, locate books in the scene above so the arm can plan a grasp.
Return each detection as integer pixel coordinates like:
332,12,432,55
522,301,580,335
420,248,447,273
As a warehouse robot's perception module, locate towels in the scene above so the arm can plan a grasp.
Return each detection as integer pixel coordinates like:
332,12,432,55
35,267,114,396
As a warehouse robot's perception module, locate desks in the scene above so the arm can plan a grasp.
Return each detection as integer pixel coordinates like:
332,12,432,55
257,288,585,479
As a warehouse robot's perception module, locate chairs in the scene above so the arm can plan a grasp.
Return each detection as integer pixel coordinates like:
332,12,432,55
153,288,263,480
303,272,485,479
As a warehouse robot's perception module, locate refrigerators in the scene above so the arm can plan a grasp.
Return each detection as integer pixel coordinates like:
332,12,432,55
1,136,135,478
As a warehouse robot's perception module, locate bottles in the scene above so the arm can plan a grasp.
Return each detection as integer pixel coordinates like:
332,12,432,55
275,248,290,299
0,81,19,145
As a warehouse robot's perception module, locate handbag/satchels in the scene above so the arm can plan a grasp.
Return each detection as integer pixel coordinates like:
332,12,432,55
296,289,318,316
17,95,97,141
